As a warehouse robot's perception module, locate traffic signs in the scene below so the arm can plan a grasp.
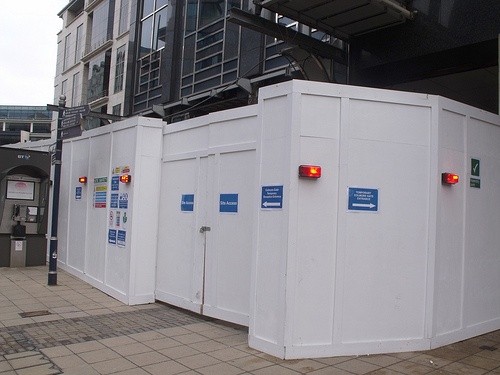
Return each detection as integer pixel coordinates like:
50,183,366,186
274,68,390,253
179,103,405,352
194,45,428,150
51,103,91,165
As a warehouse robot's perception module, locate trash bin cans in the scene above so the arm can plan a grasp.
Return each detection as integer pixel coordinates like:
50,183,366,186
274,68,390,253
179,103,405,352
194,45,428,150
9,235,27,268
